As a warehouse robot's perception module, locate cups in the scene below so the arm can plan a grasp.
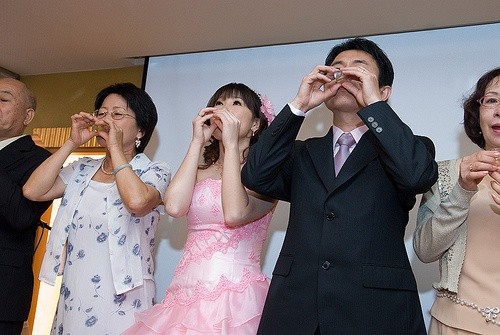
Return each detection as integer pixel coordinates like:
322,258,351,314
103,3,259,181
491,148,500,164
87,120,105,134
331,69,348,85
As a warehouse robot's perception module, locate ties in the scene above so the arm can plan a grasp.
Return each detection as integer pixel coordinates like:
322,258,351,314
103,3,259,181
333,133,356,177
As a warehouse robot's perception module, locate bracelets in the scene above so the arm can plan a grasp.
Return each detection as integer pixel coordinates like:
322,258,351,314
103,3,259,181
112,163,132,177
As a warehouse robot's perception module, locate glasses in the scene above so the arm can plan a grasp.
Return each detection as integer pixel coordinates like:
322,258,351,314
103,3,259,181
91,108,137,120
476,95,500,109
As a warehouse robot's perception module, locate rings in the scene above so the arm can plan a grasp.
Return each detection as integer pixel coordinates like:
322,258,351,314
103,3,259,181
469,162,474,171
361,68,364,73
198,113,205,117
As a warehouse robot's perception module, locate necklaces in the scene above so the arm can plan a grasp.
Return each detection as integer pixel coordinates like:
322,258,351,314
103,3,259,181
101,158,114,175
211,155,246,179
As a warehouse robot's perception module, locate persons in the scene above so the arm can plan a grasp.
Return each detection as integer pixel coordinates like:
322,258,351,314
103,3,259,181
121,81,283,335
240,35,439,335
0,66,64,335
412,66,500,335
20,80,174,335
